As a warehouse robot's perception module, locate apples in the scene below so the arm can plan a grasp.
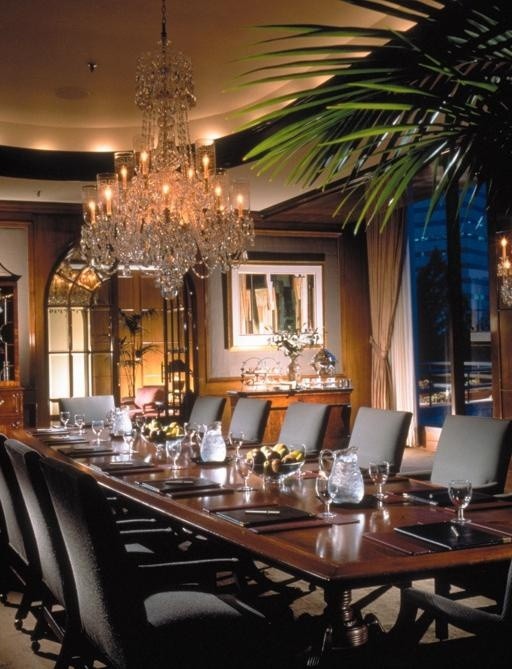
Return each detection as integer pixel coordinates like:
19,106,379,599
140,419,182,438
246,444,303,475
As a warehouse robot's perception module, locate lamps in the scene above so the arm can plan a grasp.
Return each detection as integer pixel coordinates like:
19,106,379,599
78,0,255,299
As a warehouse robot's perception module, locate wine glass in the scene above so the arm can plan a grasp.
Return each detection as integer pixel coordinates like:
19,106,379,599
448,480,472,524
315,478,338,519
368,461,390,500
59,410,255,491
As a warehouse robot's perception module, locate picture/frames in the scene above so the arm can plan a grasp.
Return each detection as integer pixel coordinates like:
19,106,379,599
225,251,328,352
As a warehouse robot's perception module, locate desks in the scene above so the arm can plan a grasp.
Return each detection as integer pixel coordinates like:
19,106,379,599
7,422,512,667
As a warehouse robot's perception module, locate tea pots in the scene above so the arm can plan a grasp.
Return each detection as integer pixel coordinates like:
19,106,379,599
105,406,133,437
319,446,365,504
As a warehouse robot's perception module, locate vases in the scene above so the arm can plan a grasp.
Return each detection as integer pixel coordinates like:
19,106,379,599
288,359,302,384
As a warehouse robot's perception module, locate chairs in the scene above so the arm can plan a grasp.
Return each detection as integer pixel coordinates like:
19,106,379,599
6,437,193,654
271,399,330,455
377,559,512,669
225,397,275,445
1,431,145,629
422,410,512,494
334,405,414,475
38,456,294,669
120,386,167,422
186,396,227,431
57,393,120,428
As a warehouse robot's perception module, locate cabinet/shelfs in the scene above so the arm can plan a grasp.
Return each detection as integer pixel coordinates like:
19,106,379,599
227,386,355,452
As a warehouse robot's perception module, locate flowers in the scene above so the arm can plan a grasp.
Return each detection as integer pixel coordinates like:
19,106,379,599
263,323,320,361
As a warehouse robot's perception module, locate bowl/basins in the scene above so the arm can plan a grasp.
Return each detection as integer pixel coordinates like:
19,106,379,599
245,460,305,483
